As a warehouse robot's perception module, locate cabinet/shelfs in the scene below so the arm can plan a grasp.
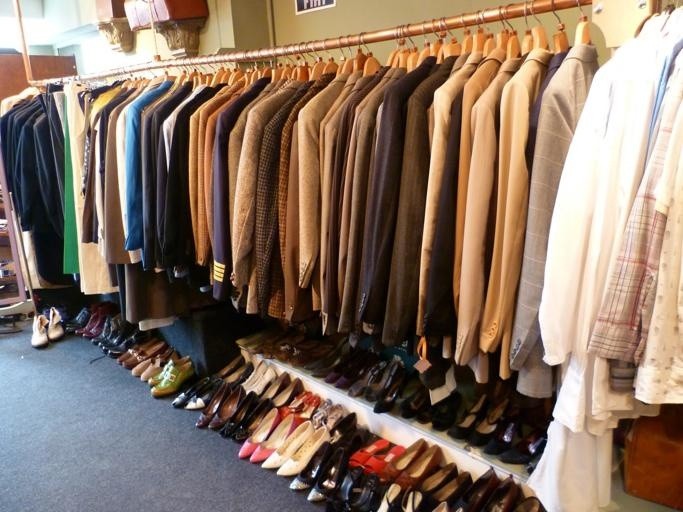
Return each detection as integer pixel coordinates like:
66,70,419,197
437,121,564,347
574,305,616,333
0,140,39,335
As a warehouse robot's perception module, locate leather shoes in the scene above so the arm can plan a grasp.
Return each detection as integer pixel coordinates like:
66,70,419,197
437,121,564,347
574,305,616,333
31,314,49,348
64,301,197,399
47,305,65,341
172,353,548,512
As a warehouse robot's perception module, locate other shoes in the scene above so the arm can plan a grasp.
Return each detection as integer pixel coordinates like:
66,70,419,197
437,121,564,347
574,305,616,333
236,324,352,354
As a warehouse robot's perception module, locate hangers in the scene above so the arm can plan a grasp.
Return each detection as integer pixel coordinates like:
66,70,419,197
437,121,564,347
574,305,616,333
11,0,681,103
